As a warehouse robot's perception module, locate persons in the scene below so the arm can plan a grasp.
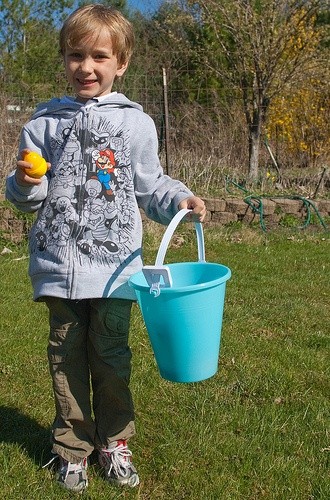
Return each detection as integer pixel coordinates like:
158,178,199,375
5,0,207,490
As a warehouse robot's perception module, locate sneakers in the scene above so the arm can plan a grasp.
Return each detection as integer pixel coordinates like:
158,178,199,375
97,439,140,487
57,455,88,490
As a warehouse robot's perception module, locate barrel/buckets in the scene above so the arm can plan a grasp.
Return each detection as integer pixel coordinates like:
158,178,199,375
128,209,231,383
